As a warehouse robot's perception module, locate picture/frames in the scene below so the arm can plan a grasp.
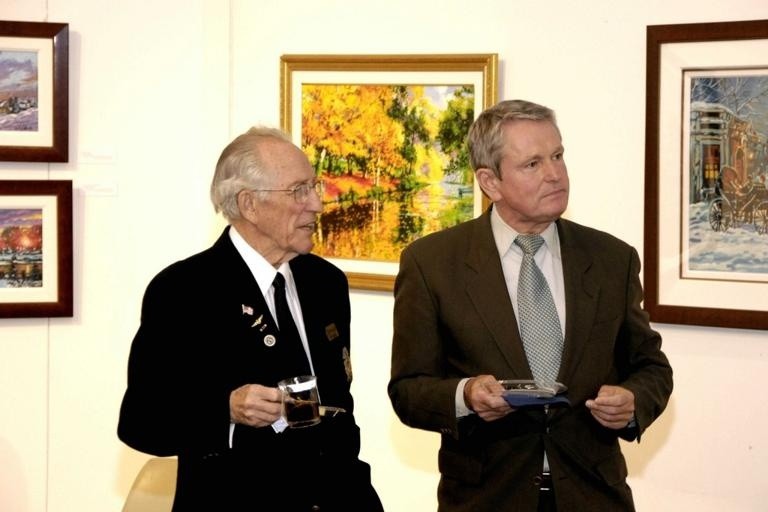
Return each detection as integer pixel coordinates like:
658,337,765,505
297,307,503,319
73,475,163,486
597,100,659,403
0,179,73,318
644,20,768,330
280,55,499,293
0,21,69,163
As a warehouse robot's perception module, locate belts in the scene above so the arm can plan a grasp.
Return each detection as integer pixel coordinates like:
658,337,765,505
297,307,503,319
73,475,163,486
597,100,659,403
538,472,551,491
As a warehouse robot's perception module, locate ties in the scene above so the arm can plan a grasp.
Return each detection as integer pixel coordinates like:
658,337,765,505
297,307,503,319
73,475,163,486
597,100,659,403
272,273,313,380
516,233,564,381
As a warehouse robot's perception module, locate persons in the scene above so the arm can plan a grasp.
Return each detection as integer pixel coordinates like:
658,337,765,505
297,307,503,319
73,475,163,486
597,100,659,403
387,96,673,512
115,127,387,511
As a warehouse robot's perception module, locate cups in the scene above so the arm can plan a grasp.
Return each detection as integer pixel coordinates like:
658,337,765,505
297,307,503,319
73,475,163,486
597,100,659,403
278,375,321,430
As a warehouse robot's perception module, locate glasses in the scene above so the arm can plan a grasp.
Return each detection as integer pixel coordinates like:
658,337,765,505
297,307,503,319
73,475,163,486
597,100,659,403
251,179,324,205
